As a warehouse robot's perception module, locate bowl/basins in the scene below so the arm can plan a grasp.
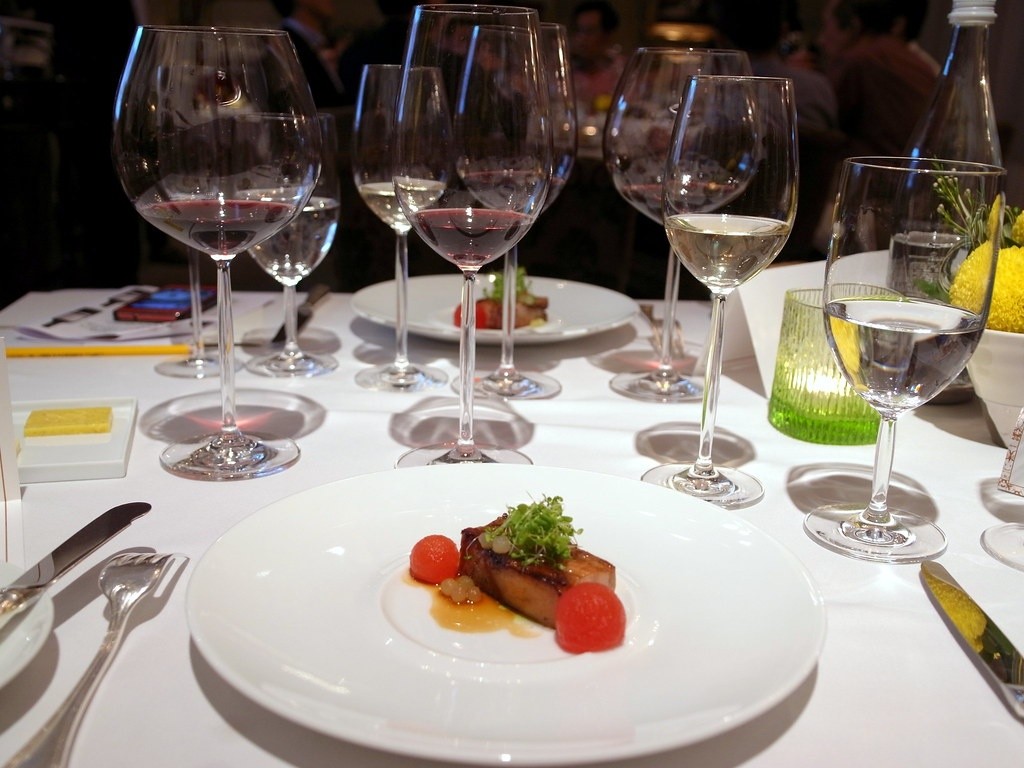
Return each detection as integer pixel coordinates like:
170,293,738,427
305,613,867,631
965,329,1024,451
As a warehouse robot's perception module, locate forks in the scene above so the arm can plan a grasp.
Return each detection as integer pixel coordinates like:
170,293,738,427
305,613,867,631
1,550,190,768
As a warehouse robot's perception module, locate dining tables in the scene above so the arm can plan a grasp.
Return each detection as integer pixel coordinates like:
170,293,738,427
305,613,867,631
0,113,1024,768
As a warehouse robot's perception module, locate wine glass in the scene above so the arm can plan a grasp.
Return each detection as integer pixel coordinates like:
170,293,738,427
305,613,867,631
243,112,344,377
153,61,245,379
981,521,1024,570
599,46,763,405
391,4,554,467
642,75,802,506
804,154,1010,561
346,62,457,392
453,24,581,398
112,21,323,481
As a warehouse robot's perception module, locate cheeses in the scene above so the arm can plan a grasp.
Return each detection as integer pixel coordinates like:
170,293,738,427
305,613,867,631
24,406,113,437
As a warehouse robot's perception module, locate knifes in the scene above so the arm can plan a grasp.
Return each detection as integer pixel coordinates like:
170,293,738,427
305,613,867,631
1,501,152,624
272,284,329,345
919,560,1024,721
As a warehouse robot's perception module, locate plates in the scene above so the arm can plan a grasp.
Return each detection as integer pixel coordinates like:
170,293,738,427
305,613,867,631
347,270,645,345
185,460,826,766
0,558,57,687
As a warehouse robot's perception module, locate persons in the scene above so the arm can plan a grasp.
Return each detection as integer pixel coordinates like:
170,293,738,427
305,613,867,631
264,0,947,285
32,1,171,290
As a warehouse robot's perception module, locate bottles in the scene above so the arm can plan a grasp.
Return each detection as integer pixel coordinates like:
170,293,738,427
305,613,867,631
883,0,1003,407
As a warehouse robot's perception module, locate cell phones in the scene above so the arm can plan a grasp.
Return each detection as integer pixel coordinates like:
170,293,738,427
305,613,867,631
113,281,222,324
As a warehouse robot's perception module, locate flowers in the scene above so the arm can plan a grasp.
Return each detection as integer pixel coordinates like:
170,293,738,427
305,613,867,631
912,157,1024,333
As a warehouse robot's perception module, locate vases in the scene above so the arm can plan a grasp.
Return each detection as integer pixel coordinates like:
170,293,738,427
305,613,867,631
958,329,1024,452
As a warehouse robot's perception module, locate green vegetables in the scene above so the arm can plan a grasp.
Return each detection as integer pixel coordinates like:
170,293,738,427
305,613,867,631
465,491,584,567
483,267,531,301
